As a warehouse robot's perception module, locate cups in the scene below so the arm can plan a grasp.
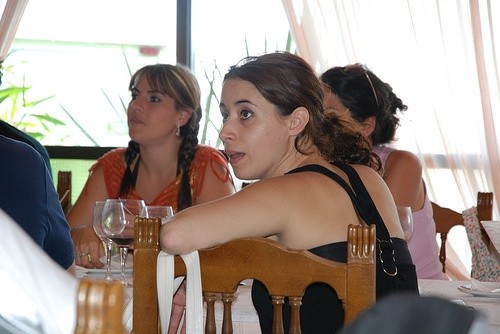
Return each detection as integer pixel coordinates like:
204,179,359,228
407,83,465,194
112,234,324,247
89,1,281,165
140,206,174,227
396,206,413,245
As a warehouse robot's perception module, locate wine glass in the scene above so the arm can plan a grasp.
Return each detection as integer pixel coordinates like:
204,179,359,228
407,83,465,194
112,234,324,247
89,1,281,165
102,199,148,286
93,199,125,282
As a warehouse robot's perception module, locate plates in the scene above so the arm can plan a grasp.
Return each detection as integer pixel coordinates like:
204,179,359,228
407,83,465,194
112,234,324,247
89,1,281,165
457,282,500,299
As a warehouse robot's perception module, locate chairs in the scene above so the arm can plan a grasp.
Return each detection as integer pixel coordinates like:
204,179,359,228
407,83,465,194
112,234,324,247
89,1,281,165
73,192,494,334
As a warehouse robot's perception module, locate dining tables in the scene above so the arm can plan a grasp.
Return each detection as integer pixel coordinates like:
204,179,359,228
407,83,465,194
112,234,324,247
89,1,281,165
69,252,500,334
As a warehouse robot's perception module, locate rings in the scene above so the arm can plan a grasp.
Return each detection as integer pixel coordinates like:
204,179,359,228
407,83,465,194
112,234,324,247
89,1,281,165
80,253,88,256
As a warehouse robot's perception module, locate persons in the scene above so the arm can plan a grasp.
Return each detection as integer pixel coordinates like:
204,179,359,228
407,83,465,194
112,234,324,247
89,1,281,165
319,64,450,279
342,295,488,334
67,63,236,269
0,120,76,274
158,52,419,334
